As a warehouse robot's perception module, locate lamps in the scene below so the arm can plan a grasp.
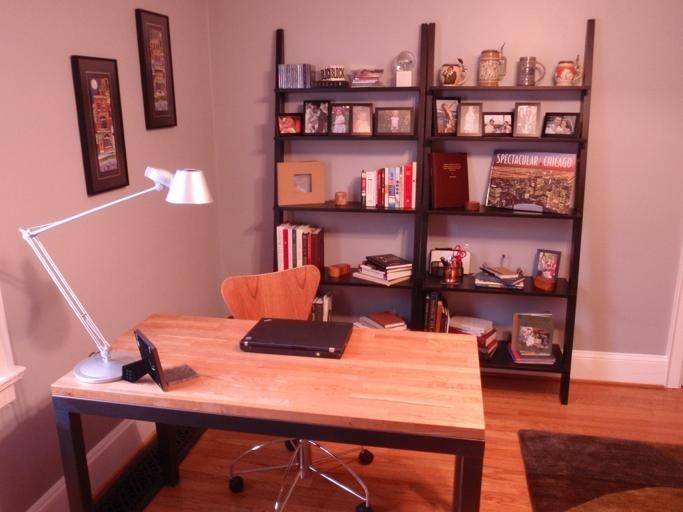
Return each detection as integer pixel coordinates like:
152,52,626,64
16,165,215,386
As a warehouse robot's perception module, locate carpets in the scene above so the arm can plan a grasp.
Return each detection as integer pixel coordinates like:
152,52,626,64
517,428,682,512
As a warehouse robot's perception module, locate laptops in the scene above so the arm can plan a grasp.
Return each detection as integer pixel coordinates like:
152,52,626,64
240,318,353,358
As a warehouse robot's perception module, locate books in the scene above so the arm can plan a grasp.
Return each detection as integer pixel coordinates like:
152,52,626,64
423,291,555,365
474,263,525,290
276,221,413,331
361,161,416,209
427,151,469,210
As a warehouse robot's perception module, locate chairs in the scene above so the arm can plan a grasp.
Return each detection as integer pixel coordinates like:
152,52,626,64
217,265,373,512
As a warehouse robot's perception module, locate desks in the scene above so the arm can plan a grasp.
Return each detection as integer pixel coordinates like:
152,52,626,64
49,308,489,512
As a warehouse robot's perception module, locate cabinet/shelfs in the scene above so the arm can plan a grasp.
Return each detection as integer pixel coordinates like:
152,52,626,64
422,18,597,408
271,24,427,327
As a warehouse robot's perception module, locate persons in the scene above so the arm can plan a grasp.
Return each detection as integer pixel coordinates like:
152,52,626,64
538,251,557,279
281,102,575,135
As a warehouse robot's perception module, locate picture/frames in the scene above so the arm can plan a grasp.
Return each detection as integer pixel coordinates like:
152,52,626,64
532,247,562,289
132,5,180,133
275,160,327,207
67,53,133,201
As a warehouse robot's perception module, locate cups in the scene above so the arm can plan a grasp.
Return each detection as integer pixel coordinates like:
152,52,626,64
552,59,583,87
440,63,470,86
477,49,507,86
516,55,546,86
